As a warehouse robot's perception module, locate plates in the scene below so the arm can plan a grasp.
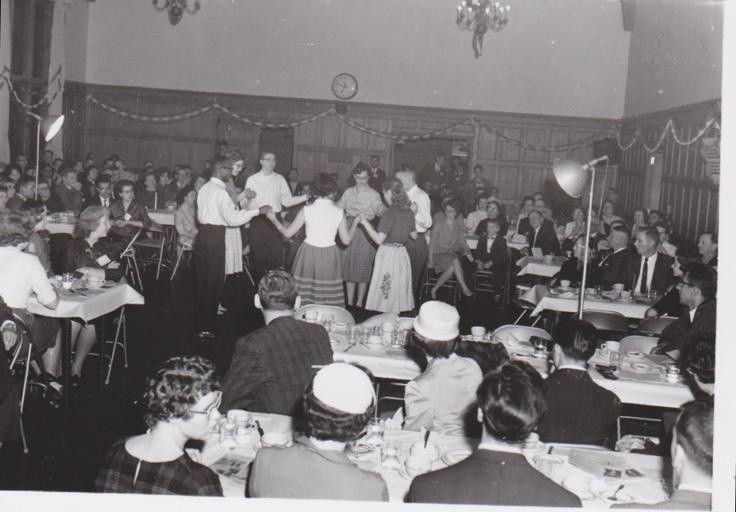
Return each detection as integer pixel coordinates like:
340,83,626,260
575,491,595,501
522,440,546,454
599,491,633,506
632,364,649,371
398,469,415,481
627,352,644,359
442,451,473,466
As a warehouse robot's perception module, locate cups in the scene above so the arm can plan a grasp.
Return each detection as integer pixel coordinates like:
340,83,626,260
62,273,72,289
380,446,400,471
560,280,570,290
562,478,590,496
471,326,485,342
260,433,288,448
304,314,408,351
405,459,432,477
226,409,251,432
614,283,624,295
87,277,106,289
667,367,681,380
600,341,619,353
523,432,539,448
365,417,384,447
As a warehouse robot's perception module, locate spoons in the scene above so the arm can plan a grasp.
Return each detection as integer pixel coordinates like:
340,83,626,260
608,485,624,500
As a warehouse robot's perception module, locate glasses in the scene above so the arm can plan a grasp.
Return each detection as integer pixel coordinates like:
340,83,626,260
355,174,370,180
98,187,111,192
220,167,233,171
189,390,223,421
122,190,135,194
676,277,695,288
236,164,245,168
262,158,277,163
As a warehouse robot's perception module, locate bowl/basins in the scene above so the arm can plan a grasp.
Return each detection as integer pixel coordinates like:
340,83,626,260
258,415,291,434
409,442,439,463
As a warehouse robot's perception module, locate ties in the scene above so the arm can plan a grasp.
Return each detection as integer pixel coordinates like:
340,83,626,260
640,257,648,294
373,169,374,175
104,199,106,207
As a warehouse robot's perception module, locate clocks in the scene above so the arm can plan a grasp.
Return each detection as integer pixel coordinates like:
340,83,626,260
332,73,358,99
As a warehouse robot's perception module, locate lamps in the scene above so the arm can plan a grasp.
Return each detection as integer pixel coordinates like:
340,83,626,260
153,0,200,25
26,108,64,203
549,154,608,322
457,1,511,58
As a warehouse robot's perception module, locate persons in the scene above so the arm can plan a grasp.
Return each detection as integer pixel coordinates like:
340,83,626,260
95,356,222,496
246,364,390,502
611,396,715,509
1,136,719,448
407,360,582,508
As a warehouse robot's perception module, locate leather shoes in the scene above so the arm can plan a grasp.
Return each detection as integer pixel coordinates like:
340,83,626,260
192,303,232,344
69,374,81,402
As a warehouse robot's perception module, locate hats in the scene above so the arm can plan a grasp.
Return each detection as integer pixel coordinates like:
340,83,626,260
412,300,461,342
313,363,375,414
